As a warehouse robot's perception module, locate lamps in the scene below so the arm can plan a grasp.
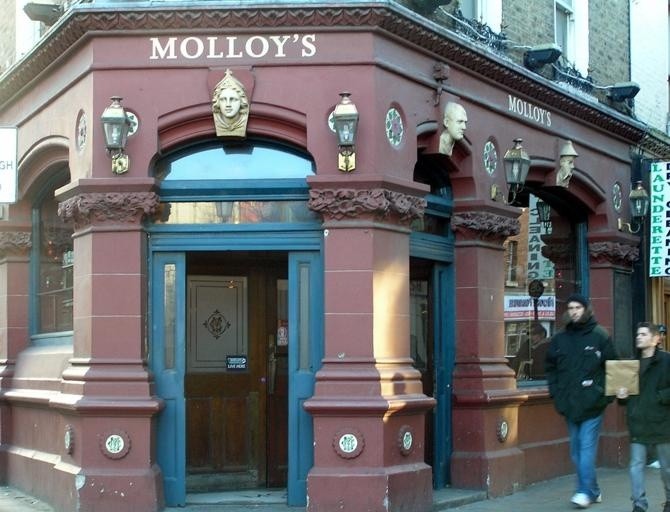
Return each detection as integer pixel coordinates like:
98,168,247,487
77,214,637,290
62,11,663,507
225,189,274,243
492,136,531,205
101,95,132,173
331,91,359,172
617,180,650,234
537,198,553,235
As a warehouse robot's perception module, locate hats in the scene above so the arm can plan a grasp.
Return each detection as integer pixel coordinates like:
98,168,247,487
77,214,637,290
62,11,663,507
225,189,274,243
567,294,590,307
657,325,666,332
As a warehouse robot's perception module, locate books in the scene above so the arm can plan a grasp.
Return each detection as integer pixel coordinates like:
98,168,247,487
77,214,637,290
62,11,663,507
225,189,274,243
605,360,640,396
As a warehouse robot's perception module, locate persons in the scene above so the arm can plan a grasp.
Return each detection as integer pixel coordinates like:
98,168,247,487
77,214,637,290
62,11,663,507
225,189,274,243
438,102,468,156
616,322,670,512
657,324,666,350
211,67,250,137
514,323,550,380
541,294,616,509
557,139,578,186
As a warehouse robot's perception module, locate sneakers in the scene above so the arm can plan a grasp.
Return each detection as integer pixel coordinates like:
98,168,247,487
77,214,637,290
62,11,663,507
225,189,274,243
663,501,670,512
632,507,644,512
571,491,601,508
